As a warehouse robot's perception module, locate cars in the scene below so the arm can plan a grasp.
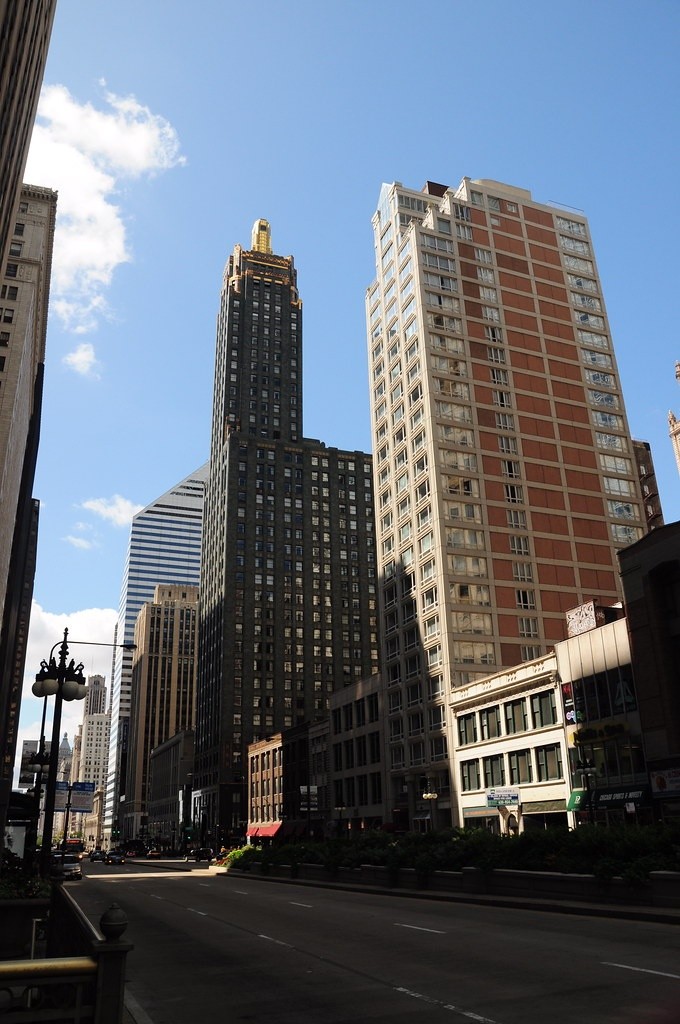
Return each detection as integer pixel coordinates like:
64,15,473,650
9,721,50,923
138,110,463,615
88,847,136,865
145,849,161,860
53,854,82,881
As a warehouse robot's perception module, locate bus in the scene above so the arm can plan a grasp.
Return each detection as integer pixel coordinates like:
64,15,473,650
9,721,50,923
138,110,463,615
59,838,86,861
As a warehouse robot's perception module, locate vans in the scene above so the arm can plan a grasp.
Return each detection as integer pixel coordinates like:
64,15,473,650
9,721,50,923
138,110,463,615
183,848,215,863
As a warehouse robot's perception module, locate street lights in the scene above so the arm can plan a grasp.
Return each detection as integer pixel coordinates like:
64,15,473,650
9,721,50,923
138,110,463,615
334,795,346,862
422,777,438,836
23,626,139,881
